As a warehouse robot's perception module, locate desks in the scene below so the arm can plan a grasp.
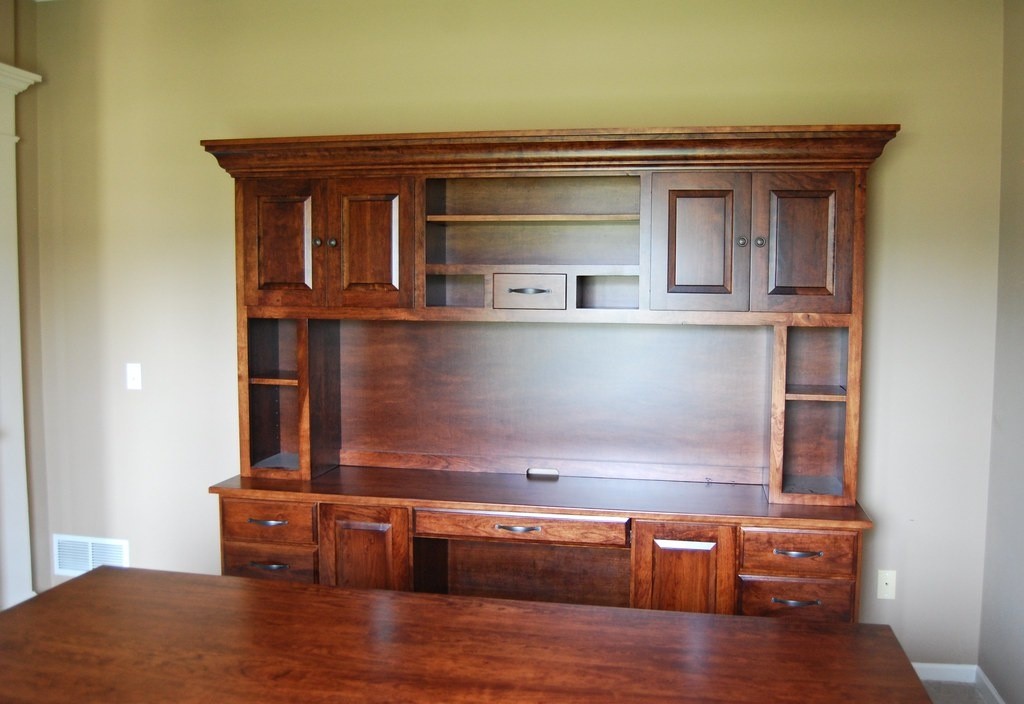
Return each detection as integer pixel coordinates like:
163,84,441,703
0,565,934,704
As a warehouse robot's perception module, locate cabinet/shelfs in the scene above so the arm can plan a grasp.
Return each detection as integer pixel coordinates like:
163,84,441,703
198,122,900,622
